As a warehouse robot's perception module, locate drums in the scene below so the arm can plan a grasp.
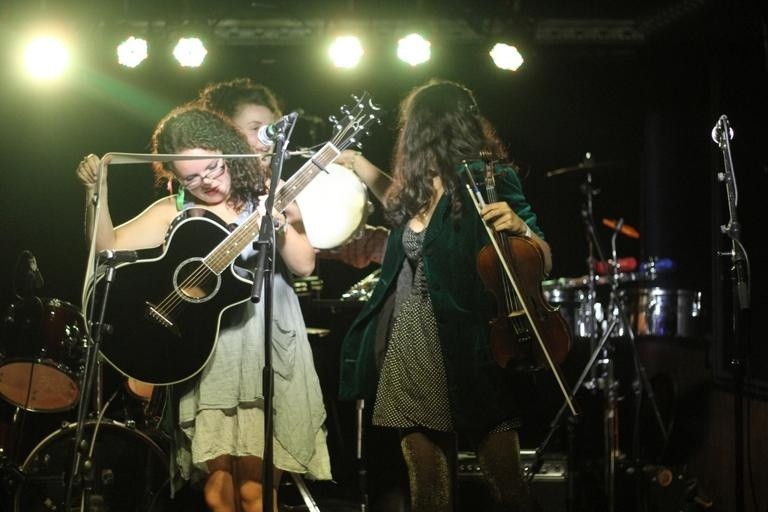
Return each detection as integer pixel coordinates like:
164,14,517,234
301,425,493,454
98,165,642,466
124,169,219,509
0,295,99,414
13,414,176,511
125,377,154,400
541,273,637,339
634,284,702,340
295,162,375,254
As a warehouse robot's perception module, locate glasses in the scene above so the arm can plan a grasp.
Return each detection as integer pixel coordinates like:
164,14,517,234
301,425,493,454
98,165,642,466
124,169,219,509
174,151,224,191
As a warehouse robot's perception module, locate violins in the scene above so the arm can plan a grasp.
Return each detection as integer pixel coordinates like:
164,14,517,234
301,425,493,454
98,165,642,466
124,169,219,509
474,149,573,372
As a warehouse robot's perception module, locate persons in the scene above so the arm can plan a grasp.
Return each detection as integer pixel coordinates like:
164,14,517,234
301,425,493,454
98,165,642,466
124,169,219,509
76,106,332,512
205,77,391,272
331,80,554,512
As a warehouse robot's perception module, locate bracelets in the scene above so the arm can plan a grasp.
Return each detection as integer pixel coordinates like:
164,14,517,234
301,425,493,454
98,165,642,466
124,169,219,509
349,151,364,171
524,221,532,240
275,211,291,237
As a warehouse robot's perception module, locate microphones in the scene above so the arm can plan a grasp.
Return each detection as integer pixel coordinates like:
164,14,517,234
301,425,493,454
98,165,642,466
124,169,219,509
711,114,734,144
25,250,45,289
257,111,298,146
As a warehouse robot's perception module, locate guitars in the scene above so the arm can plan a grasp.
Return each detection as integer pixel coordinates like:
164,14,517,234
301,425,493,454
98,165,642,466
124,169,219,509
83,90,389,386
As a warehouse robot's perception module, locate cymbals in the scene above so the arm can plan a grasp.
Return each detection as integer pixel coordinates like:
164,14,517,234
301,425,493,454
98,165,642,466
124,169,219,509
601,217,640,240
